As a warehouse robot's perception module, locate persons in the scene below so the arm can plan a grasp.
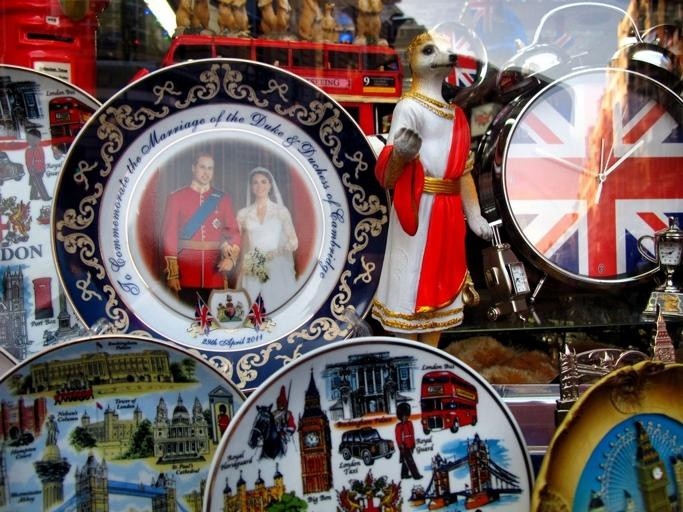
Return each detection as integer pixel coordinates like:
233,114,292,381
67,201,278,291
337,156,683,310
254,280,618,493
215,404,232,434
44,413,61,446
393,403,423,480
22,128,53,201
272,384,296,454
157,152,241,308
215,166,299,318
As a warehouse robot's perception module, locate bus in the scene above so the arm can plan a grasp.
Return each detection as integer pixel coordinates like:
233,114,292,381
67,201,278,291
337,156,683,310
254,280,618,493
45,95,96,159
420,370,480,434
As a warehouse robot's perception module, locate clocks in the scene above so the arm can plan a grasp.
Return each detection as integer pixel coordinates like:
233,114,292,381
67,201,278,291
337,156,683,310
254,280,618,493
475,3,683,290
431,3,487,91
485,244,529,324
635,223,682,318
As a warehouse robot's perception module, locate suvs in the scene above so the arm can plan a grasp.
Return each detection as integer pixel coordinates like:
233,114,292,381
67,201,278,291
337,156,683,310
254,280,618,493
336,428,395,468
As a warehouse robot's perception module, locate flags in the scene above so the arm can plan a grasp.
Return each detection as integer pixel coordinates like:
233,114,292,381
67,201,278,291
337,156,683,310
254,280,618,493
247,293,266,331
192,293,211,335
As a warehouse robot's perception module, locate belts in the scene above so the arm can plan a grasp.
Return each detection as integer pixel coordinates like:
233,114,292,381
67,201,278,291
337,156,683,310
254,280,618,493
177,238,222,250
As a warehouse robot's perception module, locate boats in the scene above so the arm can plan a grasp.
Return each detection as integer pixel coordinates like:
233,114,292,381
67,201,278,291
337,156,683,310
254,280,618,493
427,492,460,509
408,484,425,507
464,487,502,509
35,203,51,226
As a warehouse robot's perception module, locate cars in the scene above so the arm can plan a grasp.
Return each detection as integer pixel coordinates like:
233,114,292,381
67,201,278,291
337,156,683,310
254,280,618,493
0,150,26,189
